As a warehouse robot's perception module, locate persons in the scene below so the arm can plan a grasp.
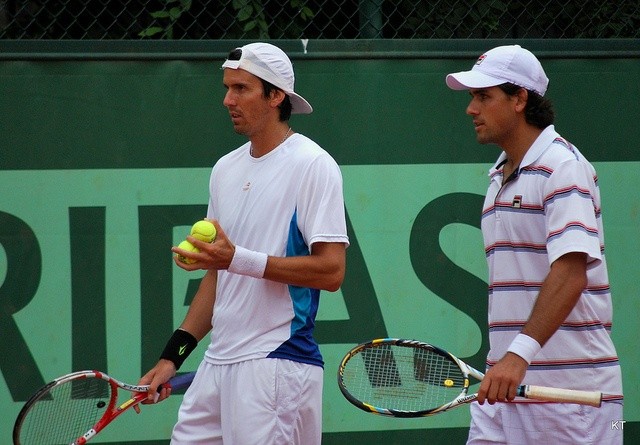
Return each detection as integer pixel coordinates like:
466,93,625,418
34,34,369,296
129,38,351,445
446,45,626,445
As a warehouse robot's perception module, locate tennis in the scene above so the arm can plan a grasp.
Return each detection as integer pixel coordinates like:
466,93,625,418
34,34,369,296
191,220,217,243
176,240,200,265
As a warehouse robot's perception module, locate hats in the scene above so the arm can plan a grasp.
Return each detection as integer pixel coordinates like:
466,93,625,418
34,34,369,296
446,43,550,97
222,42,313,116
